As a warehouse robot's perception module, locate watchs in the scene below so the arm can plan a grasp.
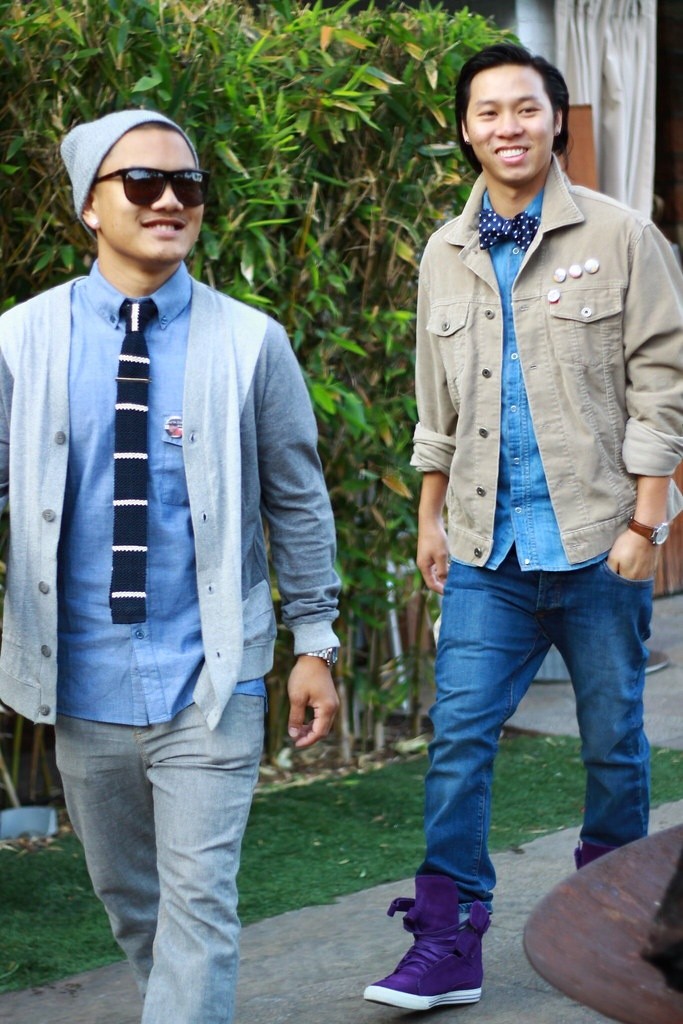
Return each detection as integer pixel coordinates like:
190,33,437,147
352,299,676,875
627,518,670,546
292,647,338,669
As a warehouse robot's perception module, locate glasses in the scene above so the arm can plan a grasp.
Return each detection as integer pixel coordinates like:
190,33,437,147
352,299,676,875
91,167,211,208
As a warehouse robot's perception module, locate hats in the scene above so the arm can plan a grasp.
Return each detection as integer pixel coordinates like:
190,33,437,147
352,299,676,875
60,110,200,239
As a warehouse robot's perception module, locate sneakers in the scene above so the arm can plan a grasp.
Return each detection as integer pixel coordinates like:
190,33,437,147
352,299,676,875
362,875,491,1010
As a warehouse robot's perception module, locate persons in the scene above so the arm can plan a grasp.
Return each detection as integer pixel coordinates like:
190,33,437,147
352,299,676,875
1,107,342,1024
363,43,683,1011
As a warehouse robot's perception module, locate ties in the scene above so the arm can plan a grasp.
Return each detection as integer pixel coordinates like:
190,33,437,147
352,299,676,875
109,298,160,624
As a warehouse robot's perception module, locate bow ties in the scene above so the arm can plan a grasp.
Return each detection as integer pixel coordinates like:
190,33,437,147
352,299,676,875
477,208,540,252
